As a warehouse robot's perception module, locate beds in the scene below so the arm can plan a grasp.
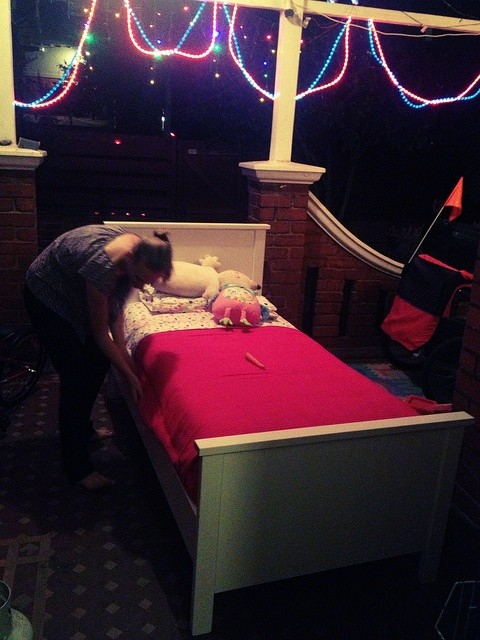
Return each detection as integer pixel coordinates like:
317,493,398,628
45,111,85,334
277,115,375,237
101,221,476,635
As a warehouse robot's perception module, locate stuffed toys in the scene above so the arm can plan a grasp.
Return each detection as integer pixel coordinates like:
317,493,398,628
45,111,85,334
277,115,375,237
203,269,262,328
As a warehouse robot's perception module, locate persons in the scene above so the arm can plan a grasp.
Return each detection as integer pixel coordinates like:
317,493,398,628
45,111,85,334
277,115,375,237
24,223,173,461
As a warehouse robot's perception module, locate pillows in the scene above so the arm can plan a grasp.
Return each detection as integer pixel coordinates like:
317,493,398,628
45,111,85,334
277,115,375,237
152,262,216,296
